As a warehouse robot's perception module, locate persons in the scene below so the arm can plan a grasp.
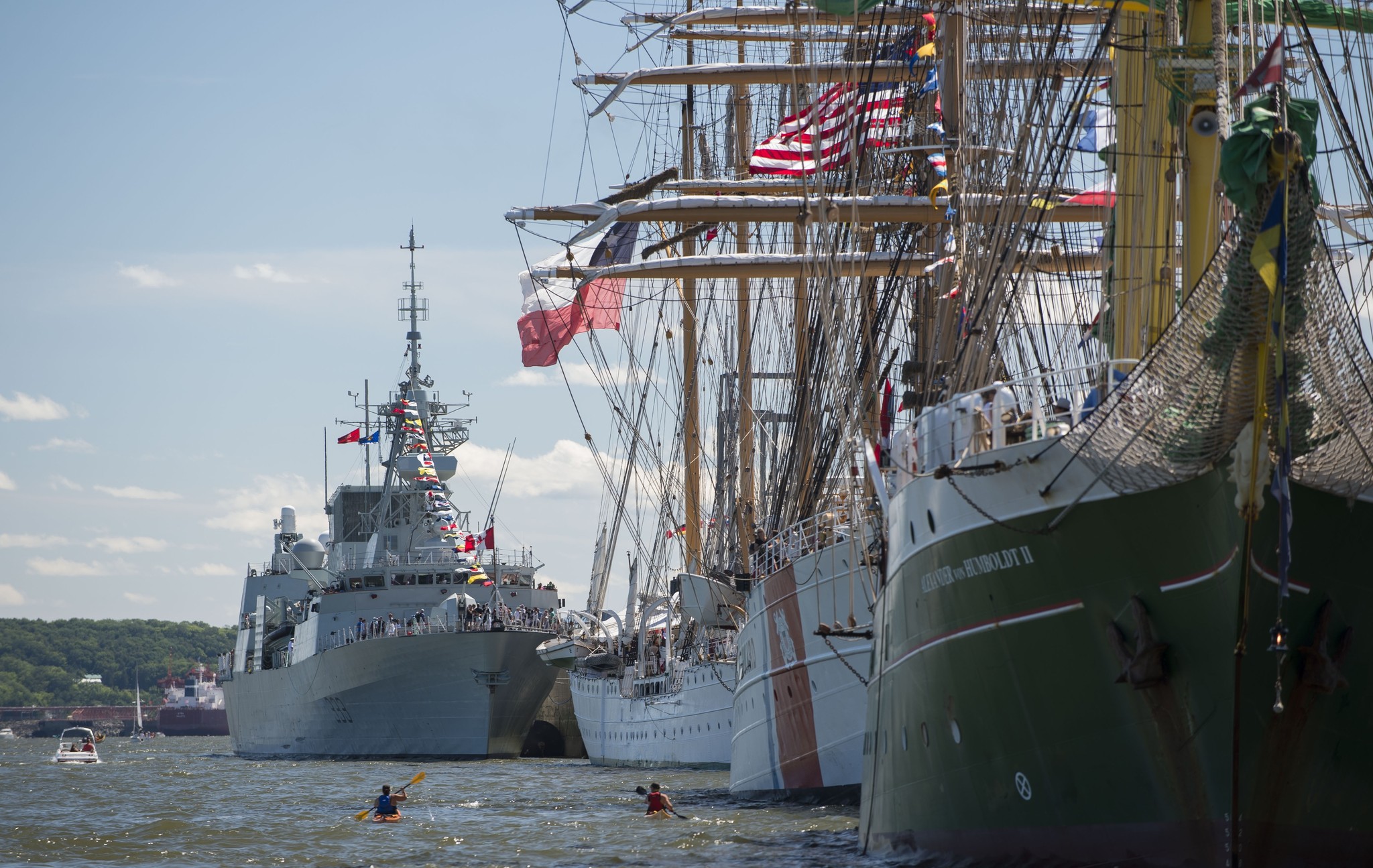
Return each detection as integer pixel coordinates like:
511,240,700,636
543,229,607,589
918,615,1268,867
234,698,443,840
532,578,556,591
374,784,407,814
464,601,574,635
391,575,413,586
596,631,660,661
980,380,1074,451
330,584,340,593
298,588,318,612
644,782,674,815
130,727,167,740
439,578,447,584
351,580,361,589
86,732,101,741
70,740,93,752
287,638,296,666
357,609,426,641
162,697,170,705
749,524,810,576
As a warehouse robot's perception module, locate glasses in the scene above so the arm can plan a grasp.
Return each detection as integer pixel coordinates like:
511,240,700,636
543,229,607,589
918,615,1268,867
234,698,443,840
545,611,547,612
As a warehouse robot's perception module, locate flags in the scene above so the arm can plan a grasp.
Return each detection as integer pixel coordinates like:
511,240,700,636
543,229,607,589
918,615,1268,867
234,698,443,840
338,427,360,444
390,398,494,583
666,513,729,539
358,430,379,445
517,220,639,370
748,23,922,178
874,377,896,469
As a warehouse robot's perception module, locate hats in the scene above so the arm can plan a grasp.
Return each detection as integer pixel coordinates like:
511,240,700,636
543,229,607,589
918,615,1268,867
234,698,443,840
477,603,480,606
359,618,363,620
516,607,520,609
482,605,485,608
378,616,382,618
652,782,660,789
373,617,378,619
290,638,294,640
383,784,391,790
421,609,424,611
1051,398,1070,409
519,604,524,607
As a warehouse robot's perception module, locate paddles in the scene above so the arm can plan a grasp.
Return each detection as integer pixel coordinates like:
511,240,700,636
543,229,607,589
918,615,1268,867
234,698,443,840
354,771,426,820
636,786,689,820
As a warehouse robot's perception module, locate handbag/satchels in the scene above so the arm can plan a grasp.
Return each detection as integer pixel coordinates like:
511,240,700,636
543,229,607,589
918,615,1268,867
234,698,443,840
362,630,366,635
473,617,476,622
370,623,373,629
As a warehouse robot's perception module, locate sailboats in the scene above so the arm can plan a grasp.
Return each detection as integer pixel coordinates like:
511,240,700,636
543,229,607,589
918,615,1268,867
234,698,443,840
129,661,151,743
532,1,1371,868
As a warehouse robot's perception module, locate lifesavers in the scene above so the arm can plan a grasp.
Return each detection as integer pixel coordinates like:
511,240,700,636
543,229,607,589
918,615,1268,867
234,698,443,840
150,732,155,738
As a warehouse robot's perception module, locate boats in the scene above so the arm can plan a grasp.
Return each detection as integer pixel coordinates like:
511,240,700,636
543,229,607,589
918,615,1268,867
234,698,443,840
159,662,229,734
221,217,576,762
55,725,106,763
641,809,673,821
372,809,402,824
